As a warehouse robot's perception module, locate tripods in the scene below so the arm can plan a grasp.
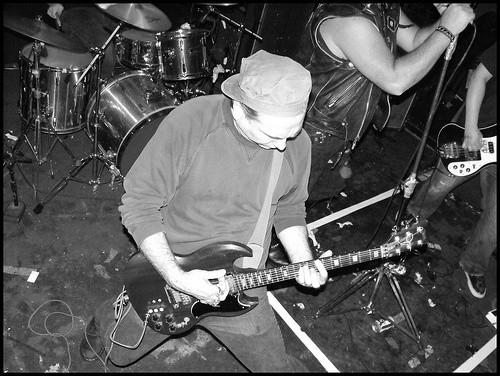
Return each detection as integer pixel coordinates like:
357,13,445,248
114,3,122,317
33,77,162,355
0,19,126,215
313,32,459,366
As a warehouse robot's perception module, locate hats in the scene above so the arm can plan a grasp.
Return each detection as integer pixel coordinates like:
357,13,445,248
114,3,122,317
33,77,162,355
220,50,313,116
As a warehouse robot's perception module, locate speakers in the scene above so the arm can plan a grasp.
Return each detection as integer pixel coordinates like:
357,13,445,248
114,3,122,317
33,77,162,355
406,51,475,147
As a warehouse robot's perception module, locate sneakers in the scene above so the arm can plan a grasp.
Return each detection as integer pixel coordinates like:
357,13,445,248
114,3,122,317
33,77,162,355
460,260,486,298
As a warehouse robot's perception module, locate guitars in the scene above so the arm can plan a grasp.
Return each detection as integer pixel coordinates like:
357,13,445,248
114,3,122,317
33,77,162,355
437,122,497,177
125,211,430,336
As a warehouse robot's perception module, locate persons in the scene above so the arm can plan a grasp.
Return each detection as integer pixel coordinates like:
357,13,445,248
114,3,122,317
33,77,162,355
79,49,331,372
268,3,475,268
407,40,497,300
45,3,125,80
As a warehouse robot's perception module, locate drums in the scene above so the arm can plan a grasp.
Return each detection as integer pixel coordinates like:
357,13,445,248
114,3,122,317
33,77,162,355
19,43,94,134
3,15,87,52
95,2,171,32
156,29,209,81
116,30,159,71
85,70,182,178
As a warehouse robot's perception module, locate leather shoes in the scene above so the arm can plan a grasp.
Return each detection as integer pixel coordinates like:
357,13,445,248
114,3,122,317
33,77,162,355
268,242,290,268
80,316,106,362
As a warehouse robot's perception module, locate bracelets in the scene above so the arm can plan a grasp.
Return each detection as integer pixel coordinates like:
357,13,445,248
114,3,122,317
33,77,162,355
435,24,455,42
